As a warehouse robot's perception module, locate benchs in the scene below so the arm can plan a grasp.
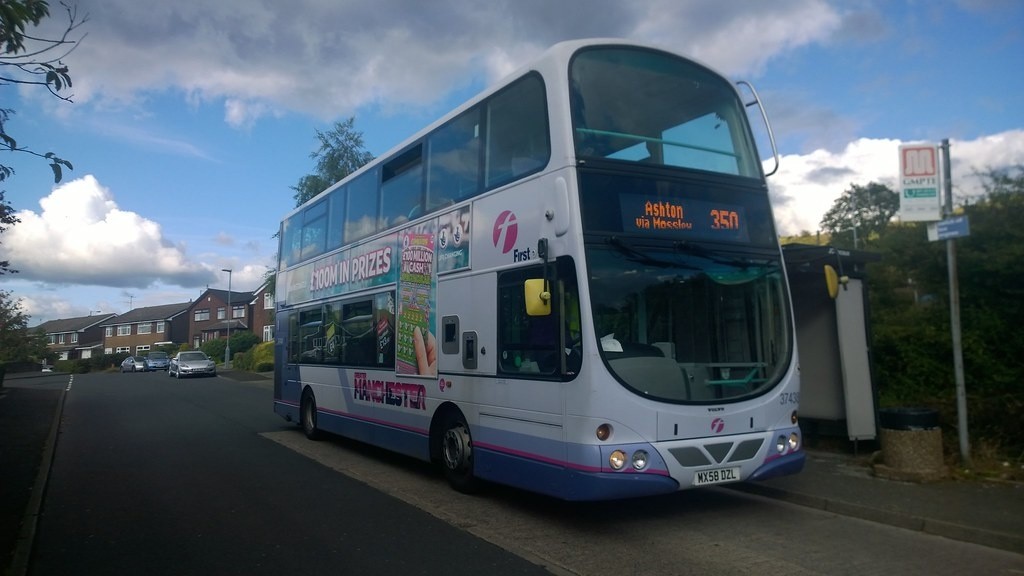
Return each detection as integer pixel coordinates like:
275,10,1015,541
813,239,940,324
384,205,421,230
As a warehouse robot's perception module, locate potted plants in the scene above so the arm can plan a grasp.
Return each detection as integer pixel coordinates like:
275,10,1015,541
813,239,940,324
870,299,1006,470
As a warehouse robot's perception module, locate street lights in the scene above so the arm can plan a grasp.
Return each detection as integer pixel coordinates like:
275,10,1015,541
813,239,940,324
222,269,232,370
90,310,101,316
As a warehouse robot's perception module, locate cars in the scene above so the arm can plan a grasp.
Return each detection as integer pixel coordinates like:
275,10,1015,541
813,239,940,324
168,351,216,379
143,352,170,372
119,357,157,373
41,366,54,373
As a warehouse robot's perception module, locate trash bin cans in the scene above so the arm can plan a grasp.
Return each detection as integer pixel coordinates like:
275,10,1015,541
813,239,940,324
867,406,949,483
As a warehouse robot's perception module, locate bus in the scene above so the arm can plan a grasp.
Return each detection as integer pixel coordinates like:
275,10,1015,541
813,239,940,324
273,36,849,502
